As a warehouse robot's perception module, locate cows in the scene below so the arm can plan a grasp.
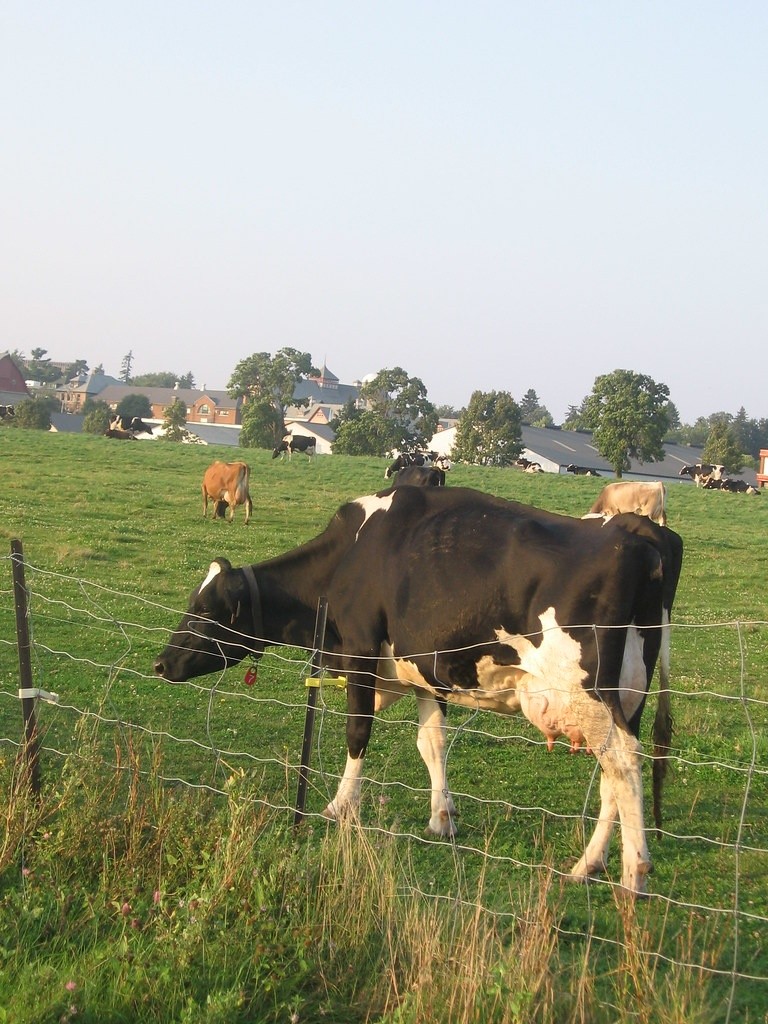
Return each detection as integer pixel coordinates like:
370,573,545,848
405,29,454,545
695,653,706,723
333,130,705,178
0,405,15,421
271,435,316,463
589,483,666,527
383,451,452,486
104,415,153,441
515,457,604,479
157,487,684,895
679,462,760,496
201,461,252,524
